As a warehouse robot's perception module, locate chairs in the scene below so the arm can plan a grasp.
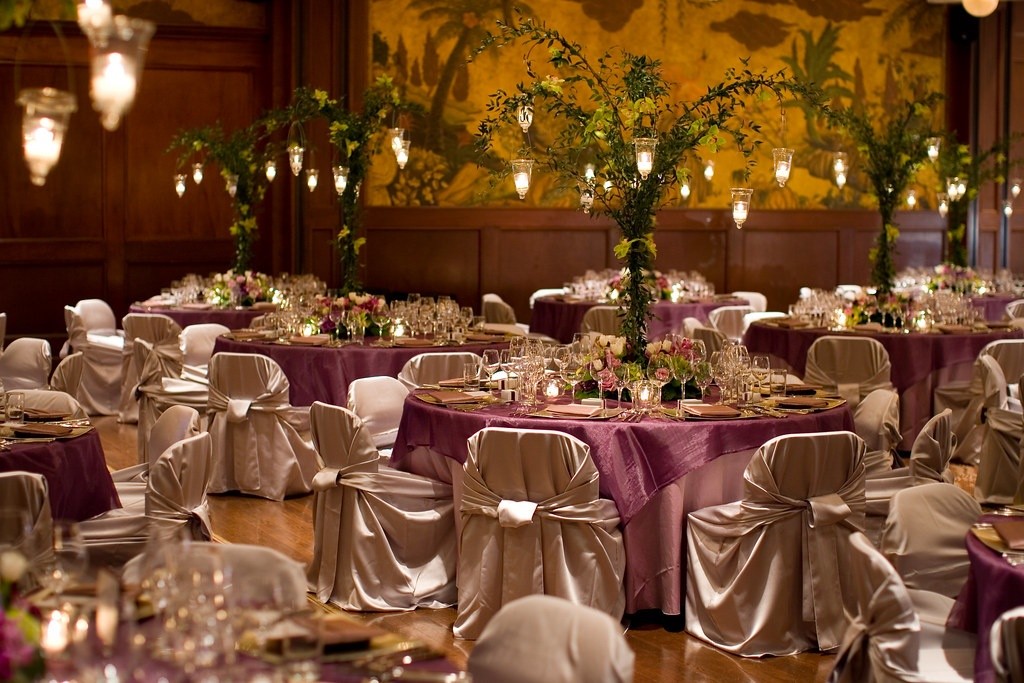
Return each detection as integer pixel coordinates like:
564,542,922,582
0,294,1024,683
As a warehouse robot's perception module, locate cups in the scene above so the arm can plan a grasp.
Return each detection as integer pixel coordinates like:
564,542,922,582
22,522,321,683
770,369,787,398
5,392,24,424
463,363,480,392
473,315,485,334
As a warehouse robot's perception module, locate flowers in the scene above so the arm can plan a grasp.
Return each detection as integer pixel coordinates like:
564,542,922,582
607,267,676,301
577,337,711,400
309,293,393,337
928,264,979,294
0,544,46,683
845,289,913,328
213,269,271,306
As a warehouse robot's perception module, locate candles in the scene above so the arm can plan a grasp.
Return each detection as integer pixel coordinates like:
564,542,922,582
174,129,411,197
508,93,1021,227
15,0,156,186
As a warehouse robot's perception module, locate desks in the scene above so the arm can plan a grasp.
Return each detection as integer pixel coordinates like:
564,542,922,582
945,506,1024,683
741,319,1024,452
209,330,511,407
129,302,275,329
0,407,124,522
0,588,472,683
534,294,726,345
391,389,857,613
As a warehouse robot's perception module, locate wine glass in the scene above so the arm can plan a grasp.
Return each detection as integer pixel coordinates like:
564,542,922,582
161,272,327,310
264,288,473,347
481,333,770,413
789,268,1024,335
562,269,714,304
